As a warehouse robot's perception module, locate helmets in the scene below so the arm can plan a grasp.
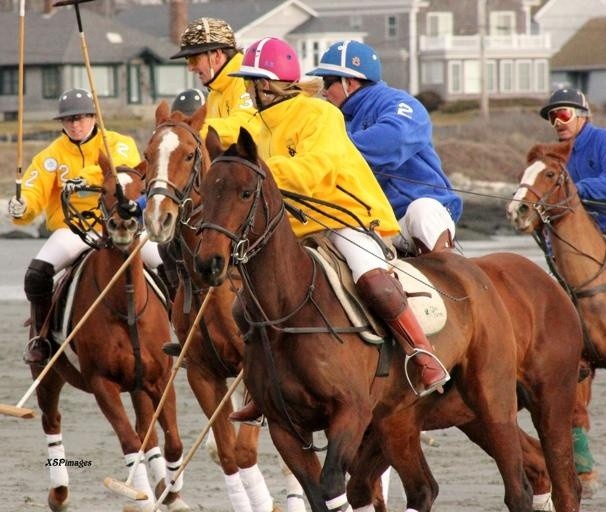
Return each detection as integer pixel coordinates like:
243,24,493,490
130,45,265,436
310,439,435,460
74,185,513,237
305,41,381,82
171,88,207,116
170,17,236,59
53,89,95,120
539,87,588,120
227,36,300,81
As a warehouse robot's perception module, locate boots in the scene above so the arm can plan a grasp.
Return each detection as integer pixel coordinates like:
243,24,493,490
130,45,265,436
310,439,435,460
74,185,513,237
229,399,261,421
384,306,445,389
24,293,50,364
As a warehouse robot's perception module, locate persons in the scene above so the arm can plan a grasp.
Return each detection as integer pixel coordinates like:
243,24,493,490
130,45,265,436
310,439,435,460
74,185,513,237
304,39,464,256
162,14,261,360
535,85,606,264
174,87,209,123
8,87,173,364
225,37,453,422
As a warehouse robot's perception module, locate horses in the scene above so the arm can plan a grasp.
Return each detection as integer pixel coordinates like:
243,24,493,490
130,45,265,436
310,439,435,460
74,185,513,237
346,252,585,512
505,138,606,500
143,98,307,512
192,126,533,512
24,148,189,512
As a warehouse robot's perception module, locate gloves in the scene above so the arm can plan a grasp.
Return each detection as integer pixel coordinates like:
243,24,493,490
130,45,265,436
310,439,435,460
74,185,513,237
63,177,85,193
8,195,26,217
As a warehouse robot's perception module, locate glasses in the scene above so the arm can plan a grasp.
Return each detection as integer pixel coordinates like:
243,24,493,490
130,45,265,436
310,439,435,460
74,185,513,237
323,74,347,88
548,107,591,126
